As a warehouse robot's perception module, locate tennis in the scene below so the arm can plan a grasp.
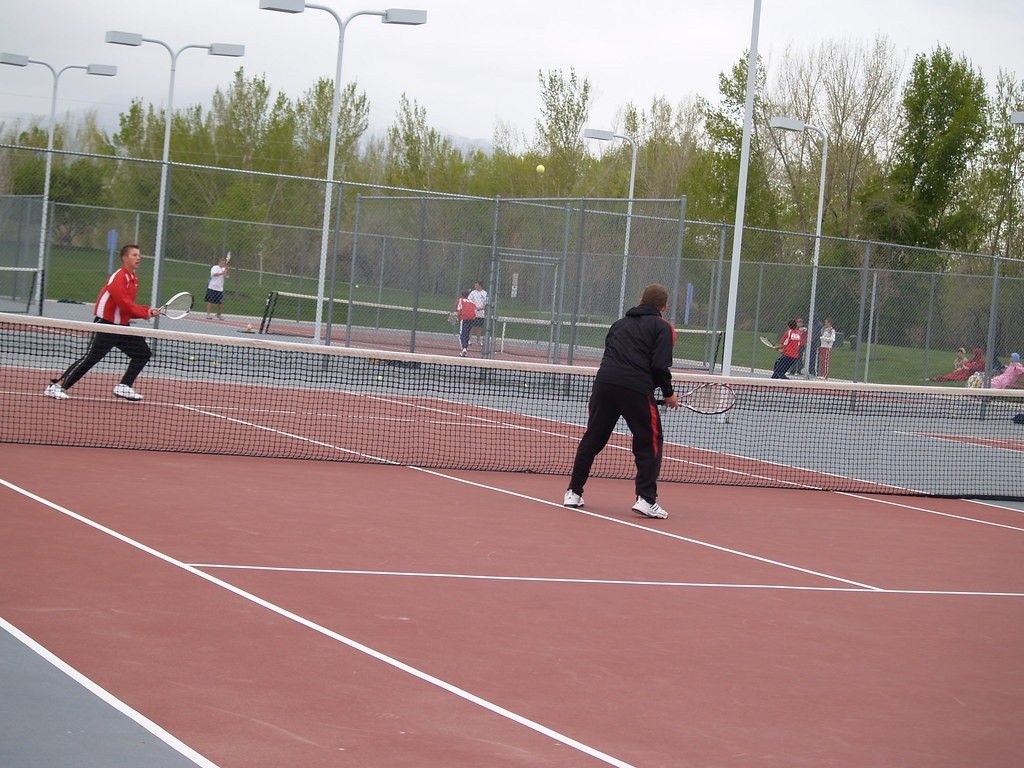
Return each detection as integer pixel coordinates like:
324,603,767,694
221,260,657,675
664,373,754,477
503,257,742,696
189,356,195,361
536,165,546,173
213,359,218,366
355,285,358,288
378,375,383,381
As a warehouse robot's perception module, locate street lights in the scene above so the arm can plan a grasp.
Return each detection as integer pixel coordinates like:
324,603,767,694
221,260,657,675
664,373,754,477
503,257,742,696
584,128,636,320
261,0,428,344
769,117,828,376
103,29,246,344
0,51,118,302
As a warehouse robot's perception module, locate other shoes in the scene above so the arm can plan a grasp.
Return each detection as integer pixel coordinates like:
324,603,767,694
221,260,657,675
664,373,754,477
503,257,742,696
784,369,830,381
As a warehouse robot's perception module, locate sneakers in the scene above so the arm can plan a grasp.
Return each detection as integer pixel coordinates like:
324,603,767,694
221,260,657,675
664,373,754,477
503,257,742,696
632,495,668,519
206,316,212,319
468,340,472,345
215,314,224,320
43,383,72,400
476,341,483,346
563,488,585,508
113,383,143,401
461,348,468,357
458,352,462,356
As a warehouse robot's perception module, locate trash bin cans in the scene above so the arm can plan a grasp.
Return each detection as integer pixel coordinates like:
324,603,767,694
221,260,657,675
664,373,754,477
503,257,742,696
849,335,857,351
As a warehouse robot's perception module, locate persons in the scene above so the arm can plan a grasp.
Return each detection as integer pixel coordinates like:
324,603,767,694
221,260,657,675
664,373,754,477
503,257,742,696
204,258,231,321
454,287,476,357
44,246,160,401
563,284,679,519
935,348,1024,389
770,318,808,379
469,280,488,347
820,318,836,380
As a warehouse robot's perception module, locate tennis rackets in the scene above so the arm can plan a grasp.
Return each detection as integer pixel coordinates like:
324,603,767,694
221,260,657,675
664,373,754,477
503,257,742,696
149,291,194,322
759,335,782,352
223,250,232,270
657,381,737,415
447,313,459,325
